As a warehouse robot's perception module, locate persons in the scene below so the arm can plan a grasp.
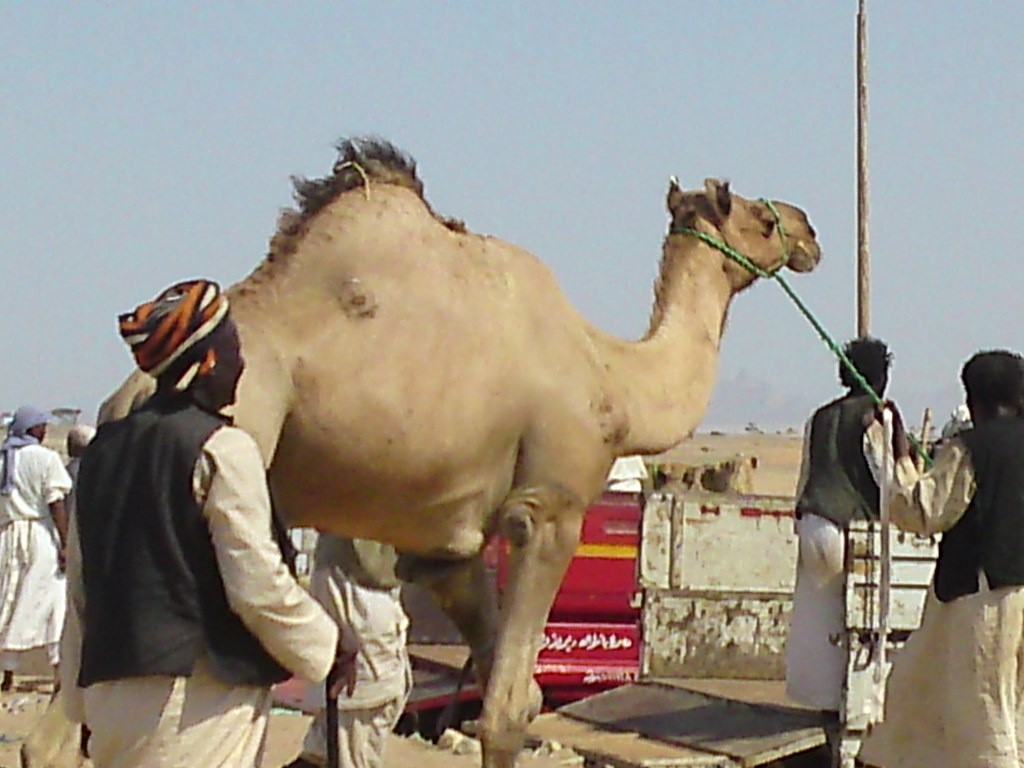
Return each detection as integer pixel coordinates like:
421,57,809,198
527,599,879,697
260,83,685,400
284,536,415,768
42,282,359,768
939,404,973,438
1,402,72,693
61,426,98,516
782,338,892,711
855,351,1023,768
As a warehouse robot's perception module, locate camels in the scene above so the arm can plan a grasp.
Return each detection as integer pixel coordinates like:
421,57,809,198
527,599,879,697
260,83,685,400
95,133,821,768
